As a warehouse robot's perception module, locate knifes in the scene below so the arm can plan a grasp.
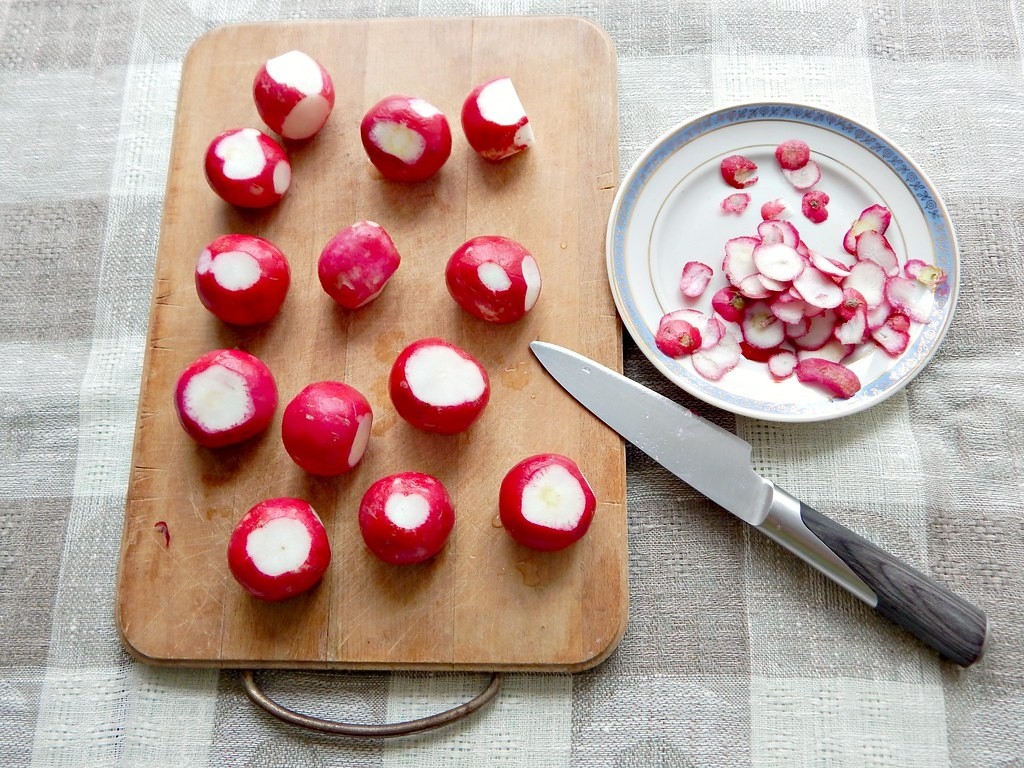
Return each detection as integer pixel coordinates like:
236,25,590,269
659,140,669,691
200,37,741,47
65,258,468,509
529,339,991,667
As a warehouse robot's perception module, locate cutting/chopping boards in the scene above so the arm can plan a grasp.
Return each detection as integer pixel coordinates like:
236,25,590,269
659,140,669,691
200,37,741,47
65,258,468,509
114,16,629,736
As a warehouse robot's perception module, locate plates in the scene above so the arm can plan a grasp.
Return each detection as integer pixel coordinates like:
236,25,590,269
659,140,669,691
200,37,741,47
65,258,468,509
605,101,961,423
